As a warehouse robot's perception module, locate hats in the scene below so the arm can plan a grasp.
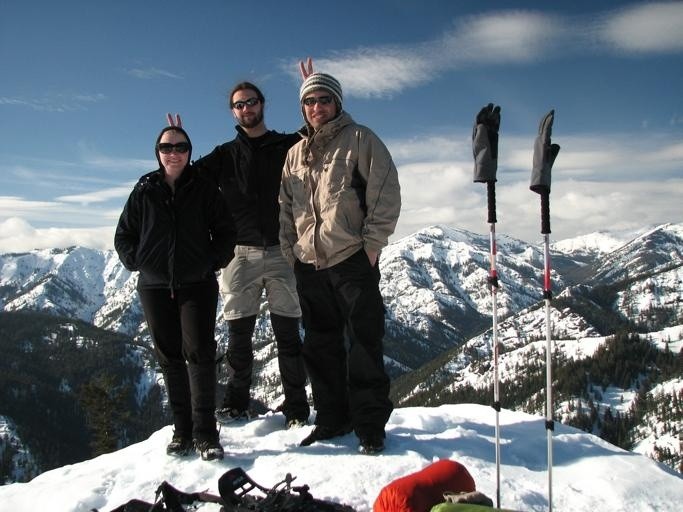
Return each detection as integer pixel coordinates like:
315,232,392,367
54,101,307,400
300,72,343,113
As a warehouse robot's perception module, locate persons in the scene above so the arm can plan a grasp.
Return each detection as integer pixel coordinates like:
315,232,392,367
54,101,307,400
114,127,235,461
277,72,401,455
166,58,313,427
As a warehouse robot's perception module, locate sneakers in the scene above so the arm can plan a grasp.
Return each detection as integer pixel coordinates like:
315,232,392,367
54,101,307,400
286,410,309,428
215,405,247,425
299,423,385,454
167,437,224,462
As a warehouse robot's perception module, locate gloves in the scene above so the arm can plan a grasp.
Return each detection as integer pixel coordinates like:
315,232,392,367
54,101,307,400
530,109,560,193
472,102,501,183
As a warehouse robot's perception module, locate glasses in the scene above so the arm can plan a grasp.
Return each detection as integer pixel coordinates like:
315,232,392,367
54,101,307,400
231,97,261,109
303,95,333,105
157,143,191,154
